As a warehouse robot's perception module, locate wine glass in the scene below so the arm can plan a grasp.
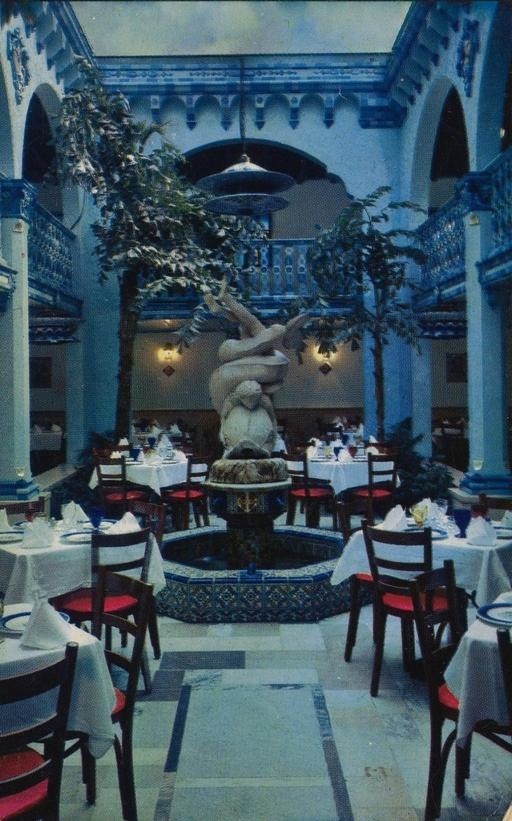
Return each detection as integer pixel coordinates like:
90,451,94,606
401,500,512,542
309,432,370,463
121,430,178,465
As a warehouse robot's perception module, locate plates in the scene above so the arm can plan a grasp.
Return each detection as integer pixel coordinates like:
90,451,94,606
1,503,119,546
476,604,512,628
3,612,71,636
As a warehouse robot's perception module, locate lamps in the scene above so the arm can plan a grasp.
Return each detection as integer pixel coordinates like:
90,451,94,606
192,58,297,219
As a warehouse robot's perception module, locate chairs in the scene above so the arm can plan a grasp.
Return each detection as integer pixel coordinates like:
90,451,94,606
331,498,510,819
28,419,468,523
1,495,164,821
